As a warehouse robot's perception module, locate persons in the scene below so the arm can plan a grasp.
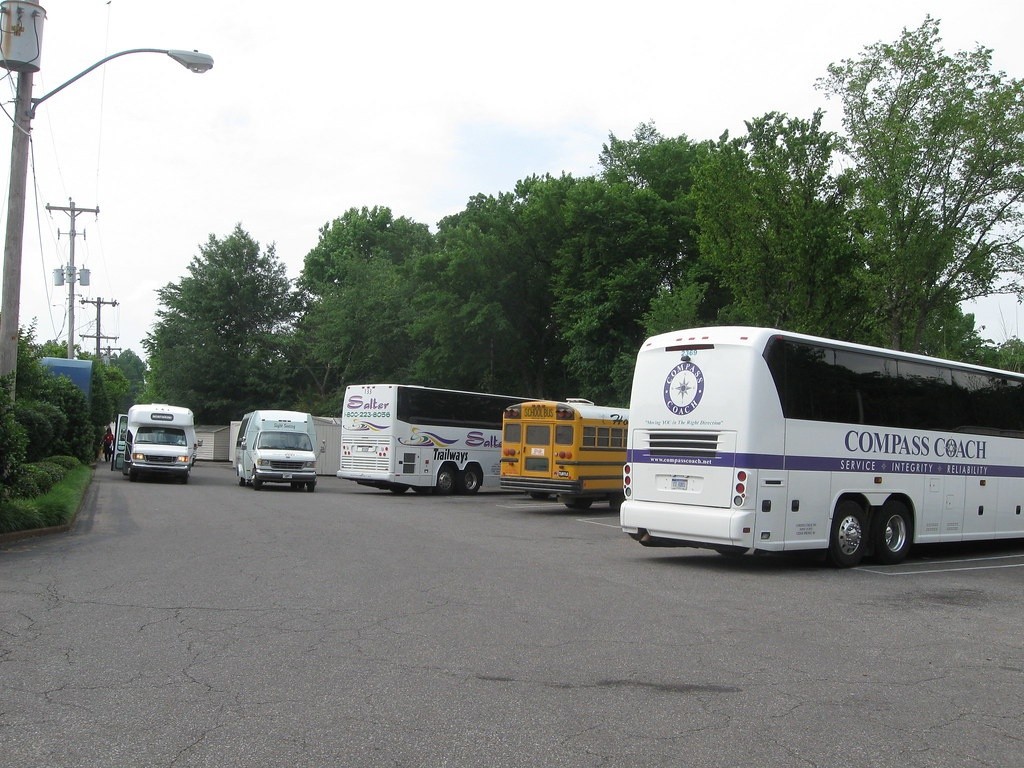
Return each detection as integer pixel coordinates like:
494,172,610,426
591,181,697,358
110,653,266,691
100,427,114,462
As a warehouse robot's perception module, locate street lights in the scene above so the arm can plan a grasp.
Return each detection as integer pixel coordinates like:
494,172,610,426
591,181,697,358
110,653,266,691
0,48,214,428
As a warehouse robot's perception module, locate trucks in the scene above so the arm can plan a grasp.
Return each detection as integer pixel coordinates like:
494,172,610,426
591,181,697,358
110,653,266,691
235,410,327,492
110,403,203,484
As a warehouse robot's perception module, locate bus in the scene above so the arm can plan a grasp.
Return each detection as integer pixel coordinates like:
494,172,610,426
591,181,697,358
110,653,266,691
336,384,594,496
499,397,628,511
620,326,1024,569
